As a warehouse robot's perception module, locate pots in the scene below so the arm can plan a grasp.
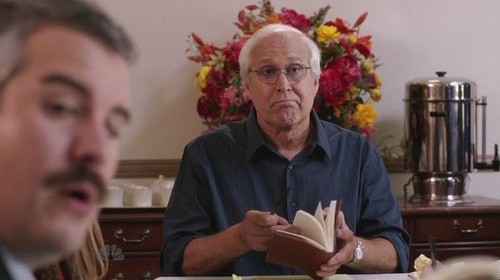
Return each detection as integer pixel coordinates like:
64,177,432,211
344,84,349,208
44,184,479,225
404,69,488,177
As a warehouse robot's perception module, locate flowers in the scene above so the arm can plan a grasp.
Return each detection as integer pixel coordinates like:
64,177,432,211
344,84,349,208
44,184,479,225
185,0,383,140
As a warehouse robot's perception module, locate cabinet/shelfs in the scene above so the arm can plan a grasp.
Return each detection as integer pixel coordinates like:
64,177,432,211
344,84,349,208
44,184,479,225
98,159,500,280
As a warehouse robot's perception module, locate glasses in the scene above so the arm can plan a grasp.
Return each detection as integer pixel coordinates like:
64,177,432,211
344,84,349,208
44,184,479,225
248,63,311,85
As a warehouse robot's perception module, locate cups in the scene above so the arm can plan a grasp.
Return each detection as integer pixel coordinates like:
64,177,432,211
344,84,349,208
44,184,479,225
107,187,123,207
155,179,174,206
127,184,153,207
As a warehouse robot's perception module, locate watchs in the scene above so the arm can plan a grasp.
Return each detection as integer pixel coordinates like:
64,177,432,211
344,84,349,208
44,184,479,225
347,236,364,265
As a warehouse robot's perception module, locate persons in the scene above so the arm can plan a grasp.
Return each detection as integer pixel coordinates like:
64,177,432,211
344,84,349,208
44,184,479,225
0,0,135,280
159,23,412,278
